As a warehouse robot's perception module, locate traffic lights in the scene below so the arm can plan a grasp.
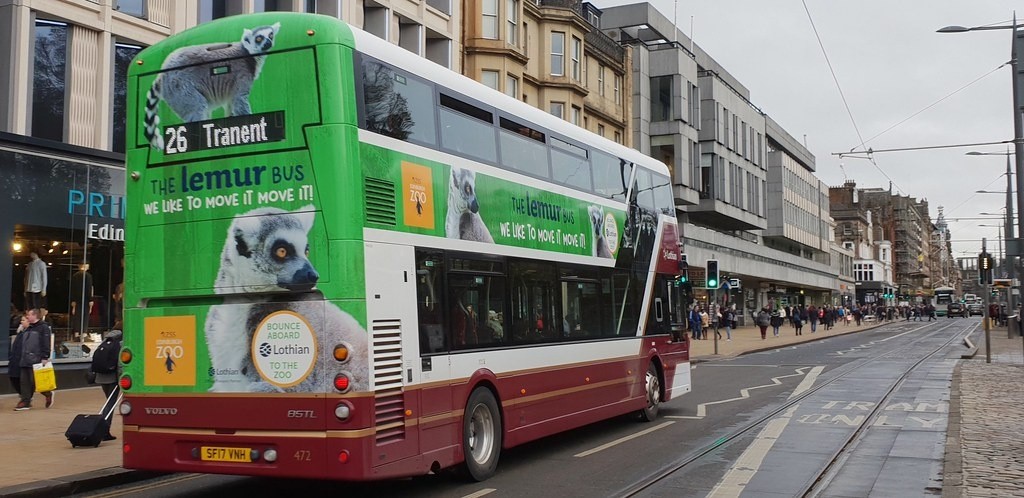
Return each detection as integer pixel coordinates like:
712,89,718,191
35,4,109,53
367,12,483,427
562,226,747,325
979,254,993,286
707,261,718,289
681,255,687,284
884,288,893,299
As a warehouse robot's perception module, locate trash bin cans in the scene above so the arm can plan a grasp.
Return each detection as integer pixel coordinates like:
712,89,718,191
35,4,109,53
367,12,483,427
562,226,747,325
1007,315,1018,339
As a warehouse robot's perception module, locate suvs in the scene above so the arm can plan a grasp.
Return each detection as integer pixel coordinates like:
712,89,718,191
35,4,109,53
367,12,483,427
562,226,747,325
947,302,969,319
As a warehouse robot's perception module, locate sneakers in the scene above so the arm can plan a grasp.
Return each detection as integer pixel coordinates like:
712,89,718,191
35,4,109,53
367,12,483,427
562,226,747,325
46,392,55,408
13,401,31,410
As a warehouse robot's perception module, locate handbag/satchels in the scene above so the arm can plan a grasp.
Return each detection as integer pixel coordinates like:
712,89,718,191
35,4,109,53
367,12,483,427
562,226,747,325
33,361,57,393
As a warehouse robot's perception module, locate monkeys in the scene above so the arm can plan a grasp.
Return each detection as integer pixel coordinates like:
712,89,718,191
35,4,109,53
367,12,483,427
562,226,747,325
588,205,614,259
202,203,369,392
445,164,496,244
143,21,281,152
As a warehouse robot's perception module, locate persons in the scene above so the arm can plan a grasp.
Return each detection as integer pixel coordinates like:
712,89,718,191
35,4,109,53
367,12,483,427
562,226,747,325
13,307,56,412
419,269,582,351
688,299,1000,343
71,260,94,341
8,313,33,401
91,320,122,440
24,248,48,309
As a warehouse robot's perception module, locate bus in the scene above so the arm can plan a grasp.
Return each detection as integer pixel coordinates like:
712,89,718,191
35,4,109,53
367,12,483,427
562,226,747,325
113,10,697,484
931,285,982,316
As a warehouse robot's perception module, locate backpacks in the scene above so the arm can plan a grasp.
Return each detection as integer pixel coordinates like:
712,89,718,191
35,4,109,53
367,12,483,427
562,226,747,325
91,335,123,374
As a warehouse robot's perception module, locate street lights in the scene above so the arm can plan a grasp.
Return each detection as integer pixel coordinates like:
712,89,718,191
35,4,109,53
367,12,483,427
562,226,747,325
935,11,1024,307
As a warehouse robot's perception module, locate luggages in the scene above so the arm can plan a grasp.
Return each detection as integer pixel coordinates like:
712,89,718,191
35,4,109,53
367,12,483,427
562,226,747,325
65,386,123,447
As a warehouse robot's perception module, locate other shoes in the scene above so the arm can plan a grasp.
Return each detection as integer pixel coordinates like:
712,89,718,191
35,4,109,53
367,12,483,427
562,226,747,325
103,432,117,441
693,315,939,342
83,336,95,342
73,336,82,342
